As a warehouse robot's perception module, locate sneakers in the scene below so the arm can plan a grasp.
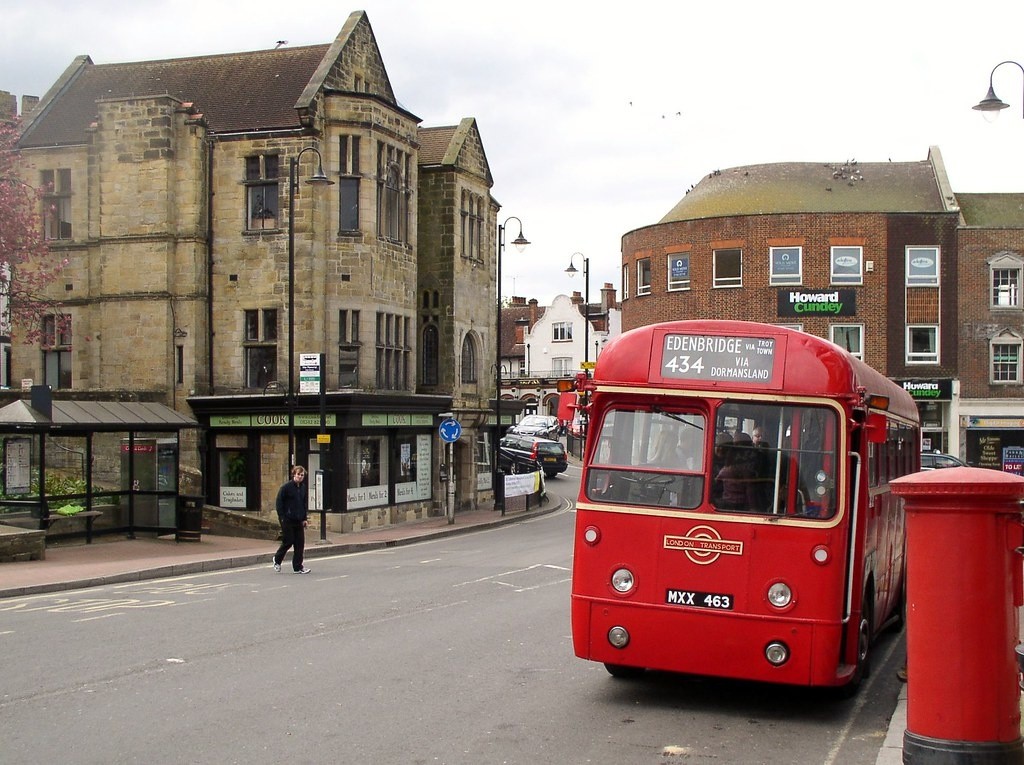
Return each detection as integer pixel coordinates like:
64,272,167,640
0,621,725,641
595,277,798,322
273,556,281,571
293,568,311,574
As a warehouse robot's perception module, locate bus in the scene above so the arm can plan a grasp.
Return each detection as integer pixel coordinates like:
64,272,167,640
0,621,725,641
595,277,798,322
570,320,921,700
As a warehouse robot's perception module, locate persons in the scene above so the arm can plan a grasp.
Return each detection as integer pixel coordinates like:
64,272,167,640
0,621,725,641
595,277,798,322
630,417,823,512
273,467,312,574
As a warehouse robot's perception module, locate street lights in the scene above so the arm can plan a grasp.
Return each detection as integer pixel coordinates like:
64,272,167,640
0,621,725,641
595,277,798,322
289,147,335,481
564,252,589,440
527,344,530,376
494,217,531,511
972,61,1023,123
595,341,598,360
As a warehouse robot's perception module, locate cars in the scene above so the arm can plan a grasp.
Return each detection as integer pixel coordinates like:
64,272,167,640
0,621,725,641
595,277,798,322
920,453,969,469
572,418,590,436
513,415,561,442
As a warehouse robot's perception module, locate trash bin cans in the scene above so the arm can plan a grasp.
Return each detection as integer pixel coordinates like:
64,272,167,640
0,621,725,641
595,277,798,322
888,466,1024,765
175,493,205,542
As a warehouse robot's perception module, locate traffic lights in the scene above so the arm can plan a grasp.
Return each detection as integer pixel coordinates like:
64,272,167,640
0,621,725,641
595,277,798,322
557,379,576,392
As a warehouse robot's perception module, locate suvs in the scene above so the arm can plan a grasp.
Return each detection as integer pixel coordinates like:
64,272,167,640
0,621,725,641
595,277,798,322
500,434,568,478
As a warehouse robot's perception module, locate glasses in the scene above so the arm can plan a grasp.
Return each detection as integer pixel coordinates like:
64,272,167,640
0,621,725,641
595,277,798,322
296,472,304,476
